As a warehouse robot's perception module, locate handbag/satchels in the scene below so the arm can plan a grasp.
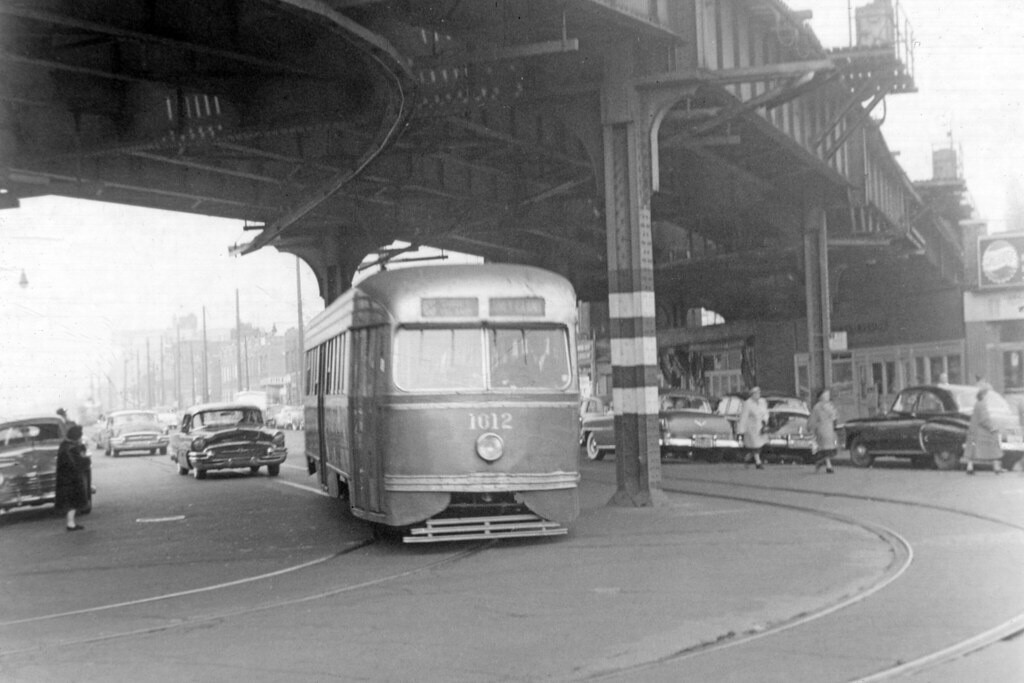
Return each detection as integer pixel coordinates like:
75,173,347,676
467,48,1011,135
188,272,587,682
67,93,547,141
761,421,769,433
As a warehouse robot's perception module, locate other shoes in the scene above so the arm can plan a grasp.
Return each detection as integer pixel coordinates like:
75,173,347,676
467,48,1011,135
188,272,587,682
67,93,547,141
815,462,819,472
756,464,764,470
67,525,84,531
826,468,834,474
743,460,748,468
995,468,1008,475
967,470,974,475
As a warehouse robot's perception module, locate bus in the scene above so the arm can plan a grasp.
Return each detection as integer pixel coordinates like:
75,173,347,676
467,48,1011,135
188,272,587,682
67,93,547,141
302,260,585,546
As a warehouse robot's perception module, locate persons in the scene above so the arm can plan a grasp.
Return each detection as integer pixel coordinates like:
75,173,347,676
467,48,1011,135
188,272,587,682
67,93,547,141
806,390,839,474
55,425,92,531
963,387,1005,475
508,331,568,387
736,386,770,470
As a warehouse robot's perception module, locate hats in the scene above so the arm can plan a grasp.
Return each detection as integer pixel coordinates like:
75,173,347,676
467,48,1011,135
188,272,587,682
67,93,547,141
57,408,67,415
749,386,759,394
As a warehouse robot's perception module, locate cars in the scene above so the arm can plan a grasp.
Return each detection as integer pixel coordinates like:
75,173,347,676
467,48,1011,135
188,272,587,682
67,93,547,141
581,395,612,444
168,402,289,478
835,385,1024,473
0,418,96,519
266,406,303,430
580,392,745,462
90,410,170,457
714,393,819,463
